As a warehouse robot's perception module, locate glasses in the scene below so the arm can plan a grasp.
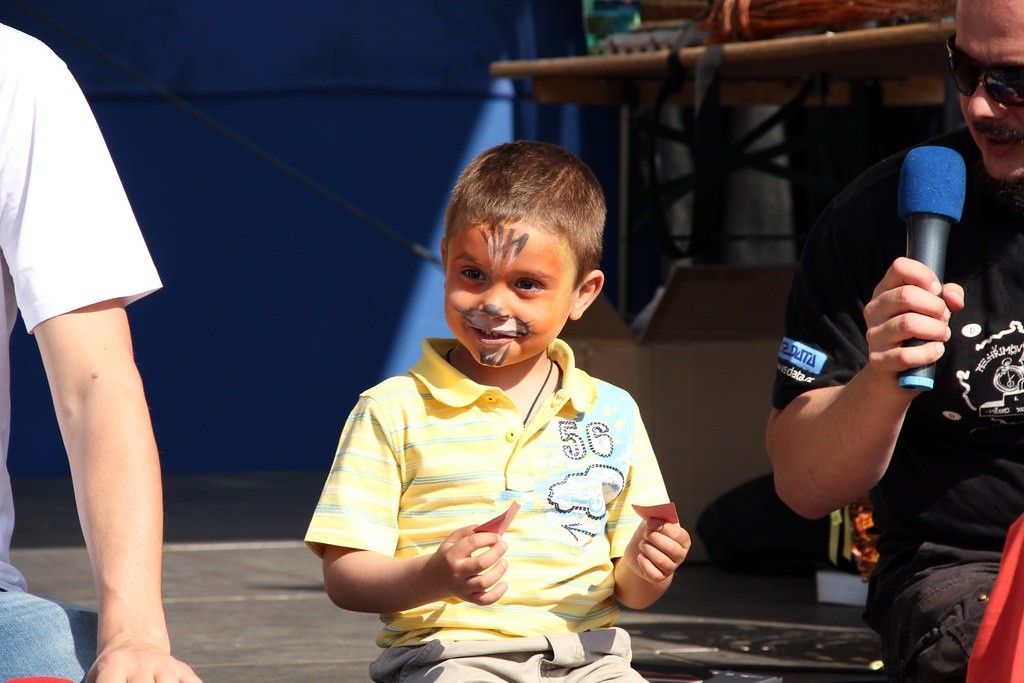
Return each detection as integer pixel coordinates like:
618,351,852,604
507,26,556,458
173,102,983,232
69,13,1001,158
946,33,1024,106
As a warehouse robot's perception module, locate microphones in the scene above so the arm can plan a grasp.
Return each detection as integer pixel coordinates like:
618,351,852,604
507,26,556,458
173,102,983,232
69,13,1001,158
898,146,966,392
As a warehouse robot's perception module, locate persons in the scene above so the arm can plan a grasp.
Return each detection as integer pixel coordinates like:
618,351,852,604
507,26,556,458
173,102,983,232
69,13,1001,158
764,1,1023,682
304,141,692,683
628,105,696,345
0,23,204,683
